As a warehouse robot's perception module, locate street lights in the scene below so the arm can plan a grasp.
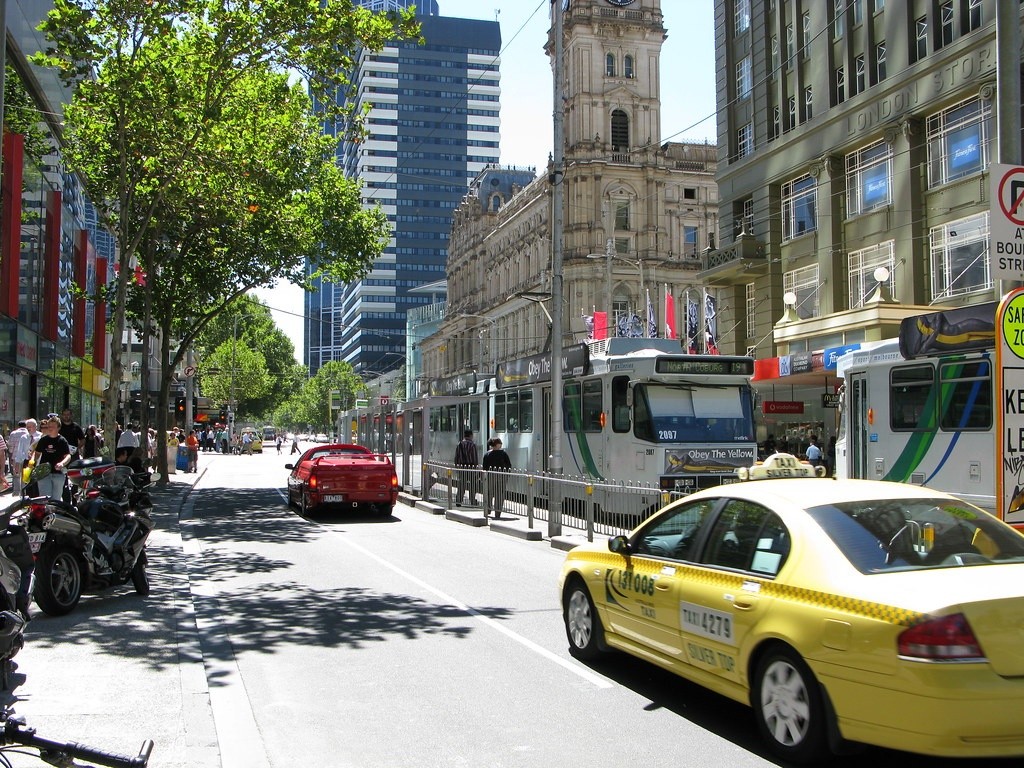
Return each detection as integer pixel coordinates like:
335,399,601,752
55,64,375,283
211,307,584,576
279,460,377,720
226,313,273,453
586,253,647,338
385,351,412,399
461,313,498,375
362,369,393,403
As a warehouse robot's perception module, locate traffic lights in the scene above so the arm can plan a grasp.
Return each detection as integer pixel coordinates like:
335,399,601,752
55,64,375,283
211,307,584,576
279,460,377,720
219,410,228,423
175,395,186,422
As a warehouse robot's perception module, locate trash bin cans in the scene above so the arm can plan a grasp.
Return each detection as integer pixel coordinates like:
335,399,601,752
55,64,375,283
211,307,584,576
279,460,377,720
176,446,188,470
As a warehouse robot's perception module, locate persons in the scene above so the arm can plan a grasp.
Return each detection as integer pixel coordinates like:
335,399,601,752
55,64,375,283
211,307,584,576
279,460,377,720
764,434,777,455
0,409,104,505
779,436,787,452
274,432,288,443
290,432,301,455
454,430,479,507
276,433,282,454
482,437,511,517
674,504,758,561
806,435,836,477
196,427,254,455
115,421,199,473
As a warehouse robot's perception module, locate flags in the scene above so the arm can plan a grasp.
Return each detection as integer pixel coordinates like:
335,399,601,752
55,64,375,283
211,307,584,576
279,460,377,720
648,297,658,338
582,314,594,338
617,314,645,338
705,293,719,354
666,291,676,339
594,311,607,339
689,299,699,355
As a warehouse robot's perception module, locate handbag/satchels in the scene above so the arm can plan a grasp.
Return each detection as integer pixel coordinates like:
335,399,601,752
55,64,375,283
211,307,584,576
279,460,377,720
293,448,296,453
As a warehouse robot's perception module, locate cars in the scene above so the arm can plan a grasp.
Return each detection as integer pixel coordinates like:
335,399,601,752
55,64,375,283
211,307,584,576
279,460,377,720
284,443,399,520
236,424,338,454
559,452,1024,768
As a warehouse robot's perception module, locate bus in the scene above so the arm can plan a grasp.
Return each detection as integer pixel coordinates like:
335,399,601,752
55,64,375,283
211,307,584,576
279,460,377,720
428,289,761,528
833,337,1024,510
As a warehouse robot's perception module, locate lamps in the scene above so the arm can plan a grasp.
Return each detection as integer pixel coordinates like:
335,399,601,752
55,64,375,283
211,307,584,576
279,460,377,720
873,265,891,282
784,291,796,304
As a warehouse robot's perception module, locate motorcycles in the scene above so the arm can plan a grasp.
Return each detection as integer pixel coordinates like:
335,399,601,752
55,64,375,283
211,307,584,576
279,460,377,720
0,455,161,691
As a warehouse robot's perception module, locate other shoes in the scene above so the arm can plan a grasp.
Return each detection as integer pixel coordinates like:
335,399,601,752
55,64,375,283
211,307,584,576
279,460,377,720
471,499,477,505
456,504,461,507
